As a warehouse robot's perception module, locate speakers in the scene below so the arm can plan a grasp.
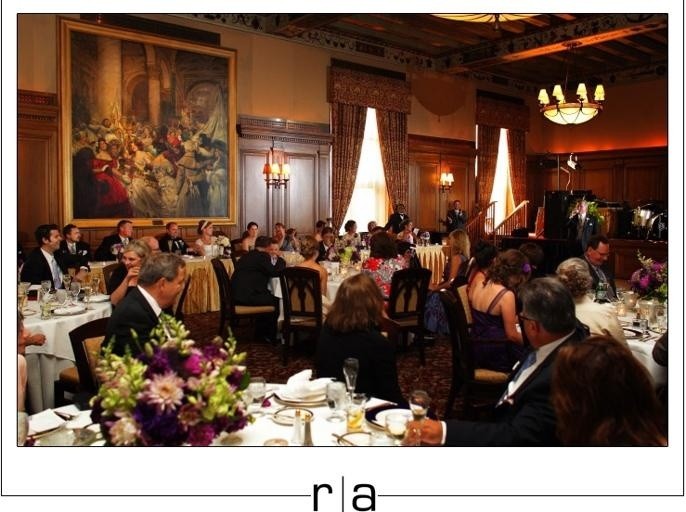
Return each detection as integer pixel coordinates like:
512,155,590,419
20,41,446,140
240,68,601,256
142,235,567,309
544,190,570,238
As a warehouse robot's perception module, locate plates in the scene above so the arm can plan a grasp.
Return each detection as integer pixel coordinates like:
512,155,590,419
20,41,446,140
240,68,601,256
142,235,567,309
338,432,374,447
362,403,429,435
271,407,315,426
270,388,328,406
66,409,96,429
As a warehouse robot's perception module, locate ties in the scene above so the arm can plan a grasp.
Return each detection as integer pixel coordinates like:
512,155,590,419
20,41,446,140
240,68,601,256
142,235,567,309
52,259,61,288
513,352,536,381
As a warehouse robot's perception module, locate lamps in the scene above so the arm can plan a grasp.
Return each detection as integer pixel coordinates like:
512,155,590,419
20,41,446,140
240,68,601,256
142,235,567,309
430,13,540,31
567,153,581,170
439,172,455,193
536,42,606,127
266,163,290,188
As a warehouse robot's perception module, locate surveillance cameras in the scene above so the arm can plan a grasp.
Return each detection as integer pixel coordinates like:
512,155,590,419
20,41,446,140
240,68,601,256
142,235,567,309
568,160,577,170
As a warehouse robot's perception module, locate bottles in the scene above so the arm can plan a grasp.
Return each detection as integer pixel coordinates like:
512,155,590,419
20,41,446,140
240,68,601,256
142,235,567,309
212,239,220,258
288,409,301,446
303,412,315,446
218,239,226,260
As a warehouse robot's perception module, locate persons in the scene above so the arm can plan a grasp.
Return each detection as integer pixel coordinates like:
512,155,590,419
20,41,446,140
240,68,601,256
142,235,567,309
72,116,229,218
17,200,668,447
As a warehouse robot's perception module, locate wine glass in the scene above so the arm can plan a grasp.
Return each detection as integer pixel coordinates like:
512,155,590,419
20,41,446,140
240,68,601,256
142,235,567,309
243,376,266,418
17,267,103,321
323,381,346,422
384,412,411,446
343,357,359,410
406,388,430,447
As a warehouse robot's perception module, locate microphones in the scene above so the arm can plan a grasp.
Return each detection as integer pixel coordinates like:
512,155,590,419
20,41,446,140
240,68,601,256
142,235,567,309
573,210,579,214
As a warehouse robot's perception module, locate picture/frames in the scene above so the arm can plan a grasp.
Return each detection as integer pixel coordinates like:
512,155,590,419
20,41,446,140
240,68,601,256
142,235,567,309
54,15,239,229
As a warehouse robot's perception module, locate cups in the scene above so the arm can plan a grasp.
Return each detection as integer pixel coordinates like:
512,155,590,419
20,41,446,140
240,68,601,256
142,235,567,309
345,399,365,432
611,290,668,342
